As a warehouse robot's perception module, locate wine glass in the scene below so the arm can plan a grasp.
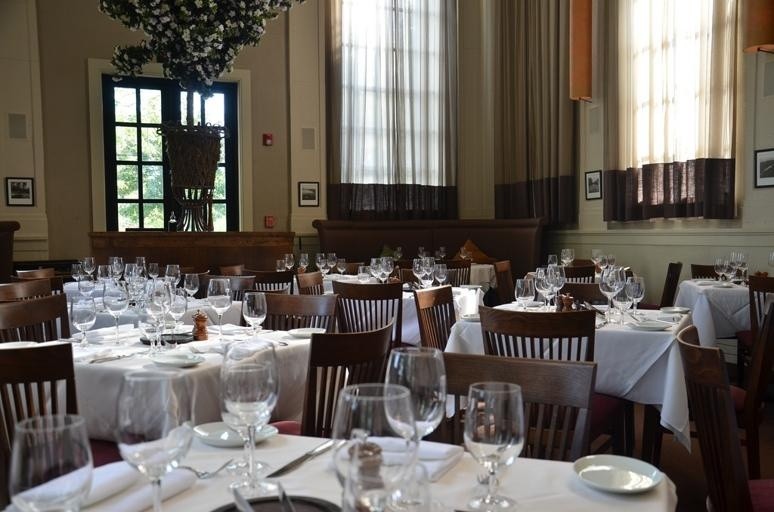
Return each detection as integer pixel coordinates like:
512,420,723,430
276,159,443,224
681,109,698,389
714,251,750,286
460,377,525,511
108,368,195,511
67,256,200,356
276,251,345,278
240,291,267,339
512,265,646,329
215,339,286,493
355,252,447,287
206,276,234,342
392,245,467,261
380,346,448,471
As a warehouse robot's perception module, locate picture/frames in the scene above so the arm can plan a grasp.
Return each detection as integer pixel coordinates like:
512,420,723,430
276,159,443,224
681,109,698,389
5,176,34,207
584,169,601,200
298,181,319,207
754,148,774,188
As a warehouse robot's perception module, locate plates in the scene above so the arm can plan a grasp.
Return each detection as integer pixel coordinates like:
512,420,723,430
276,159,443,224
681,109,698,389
661,305,690,315
571,453,665,495
461,313,482,322
208,493,342,511
460,284,482,289
285,327,326,338
193,417,280,450
143,352,204,369
625,318,672,333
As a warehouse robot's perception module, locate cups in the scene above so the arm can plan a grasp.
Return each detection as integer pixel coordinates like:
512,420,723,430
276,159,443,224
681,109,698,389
543,248,616,269
769,253,774,268
8,414,92,512
329,379,431,511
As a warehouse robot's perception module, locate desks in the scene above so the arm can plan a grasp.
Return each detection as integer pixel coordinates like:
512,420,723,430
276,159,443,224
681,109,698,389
312,216,551,301
88,230,296,289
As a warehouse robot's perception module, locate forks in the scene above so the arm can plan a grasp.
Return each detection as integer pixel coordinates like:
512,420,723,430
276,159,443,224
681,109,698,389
169,458,234,482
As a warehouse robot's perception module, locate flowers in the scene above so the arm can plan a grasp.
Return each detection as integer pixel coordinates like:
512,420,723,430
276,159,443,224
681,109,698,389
97,0,309,130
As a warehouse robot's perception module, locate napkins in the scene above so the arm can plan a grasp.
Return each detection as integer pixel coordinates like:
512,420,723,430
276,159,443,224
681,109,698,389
365,432,465,480
12,458,138,509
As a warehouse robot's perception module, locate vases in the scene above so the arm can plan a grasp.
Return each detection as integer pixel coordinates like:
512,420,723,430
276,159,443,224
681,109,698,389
156,121,228,231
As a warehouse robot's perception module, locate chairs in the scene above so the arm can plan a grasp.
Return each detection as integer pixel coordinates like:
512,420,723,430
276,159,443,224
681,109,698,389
677,325,773,512
0,264,772,369
478,303,616,461
299,318,394,440
399,351,598,462
0,343,80,511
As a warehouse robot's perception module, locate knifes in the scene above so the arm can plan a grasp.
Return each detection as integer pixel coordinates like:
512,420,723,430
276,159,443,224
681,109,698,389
91,354,138,363
274,481,297,511
262,438,332,480
232,486,256,512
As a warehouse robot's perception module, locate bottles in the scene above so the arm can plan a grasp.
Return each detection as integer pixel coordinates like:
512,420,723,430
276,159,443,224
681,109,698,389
190,308,208,340
